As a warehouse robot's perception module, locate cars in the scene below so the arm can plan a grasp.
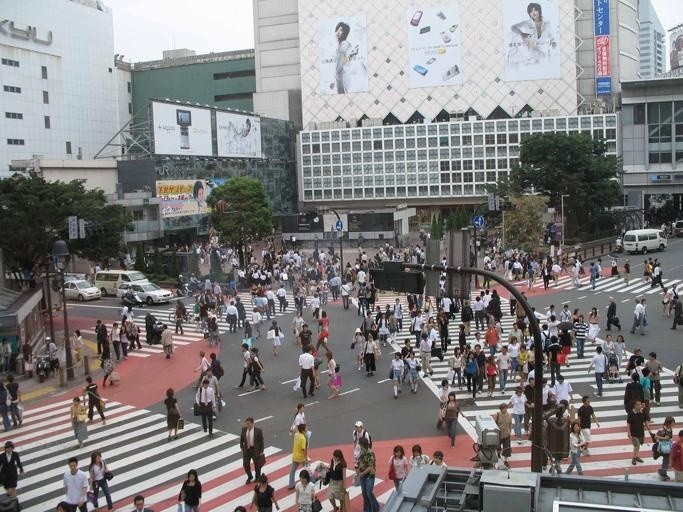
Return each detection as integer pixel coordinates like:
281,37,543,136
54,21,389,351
116,281,173,305
674,220,683,236
59,279,102,302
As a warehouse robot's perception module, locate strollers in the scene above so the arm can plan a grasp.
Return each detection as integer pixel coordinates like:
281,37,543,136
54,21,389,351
605,351,621,383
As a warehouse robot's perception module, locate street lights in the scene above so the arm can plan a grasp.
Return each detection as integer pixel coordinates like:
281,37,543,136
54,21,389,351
560,194,570,244
289,236,296,250
312,207,343,284
36,225,74,378
472,198,504,279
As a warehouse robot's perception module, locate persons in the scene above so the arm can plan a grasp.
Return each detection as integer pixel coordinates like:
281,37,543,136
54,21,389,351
181,181,208,216
318,20,359,95
508,2,556,66
225,119,252,141
670,35,683,69
1,194,683,512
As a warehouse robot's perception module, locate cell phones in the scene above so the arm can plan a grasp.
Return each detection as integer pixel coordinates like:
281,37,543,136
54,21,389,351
410,9,460,81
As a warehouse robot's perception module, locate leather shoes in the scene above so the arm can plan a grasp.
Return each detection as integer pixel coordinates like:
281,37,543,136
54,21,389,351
253,475,260,482
245,475,252,484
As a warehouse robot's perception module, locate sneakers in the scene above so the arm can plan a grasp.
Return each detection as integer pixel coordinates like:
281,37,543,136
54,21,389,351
303,391,315,398
451,374,517,401
503,460,512,468
233,384,265,391
631,456,643,465
167,426,213,441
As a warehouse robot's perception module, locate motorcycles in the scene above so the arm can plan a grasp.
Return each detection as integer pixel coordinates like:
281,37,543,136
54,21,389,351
615,235,622,253
30,353,58,382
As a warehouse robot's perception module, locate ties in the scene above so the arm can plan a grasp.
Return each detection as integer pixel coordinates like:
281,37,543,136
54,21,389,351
246,431,250,449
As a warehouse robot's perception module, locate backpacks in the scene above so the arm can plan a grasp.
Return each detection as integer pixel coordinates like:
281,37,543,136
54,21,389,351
542,330,552,353
353,428,372,448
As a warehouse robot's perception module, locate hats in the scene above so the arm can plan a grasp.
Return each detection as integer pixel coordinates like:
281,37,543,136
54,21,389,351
499,403,509,410
514,386,525,392
354,420,364,427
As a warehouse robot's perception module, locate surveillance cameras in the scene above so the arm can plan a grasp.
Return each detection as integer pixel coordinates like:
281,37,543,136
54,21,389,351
476,415,500,464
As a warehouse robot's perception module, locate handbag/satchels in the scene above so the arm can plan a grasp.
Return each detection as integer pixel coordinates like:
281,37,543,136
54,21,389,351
103,470,113,481
299,467,361,512
98,399,105,412
610,316,683,326
5,399,11,406
85,490,96,504
177,398,222,428
255,454,265,466
388,464,395,480
267,329,284,339
313,357,340,390
350,322,455,382
602,370,607,379
651,439,672,460
287,423,295,432
199,367,224,381
180,489,186,501
252,494,257,506
16,405,23,418
76,427,87,442
246,359,262,372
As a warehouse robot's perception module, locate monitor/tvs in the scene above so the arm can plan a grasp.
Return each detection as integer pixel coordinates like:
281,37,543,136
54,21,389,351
176,109,192,126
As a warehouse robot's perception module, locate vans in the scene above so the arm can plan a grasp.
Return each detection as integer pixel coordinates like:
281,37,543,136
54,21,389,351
622,229,667,254
94,270,149,297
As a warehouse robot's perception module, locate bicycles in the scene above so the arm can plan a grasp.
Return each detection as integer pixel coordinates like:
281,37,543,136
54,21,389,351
168,311,195,324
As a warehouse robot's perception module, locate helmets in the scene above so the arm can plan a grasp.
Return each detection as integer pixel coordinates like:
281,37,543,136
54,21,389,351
44,336,51,343
